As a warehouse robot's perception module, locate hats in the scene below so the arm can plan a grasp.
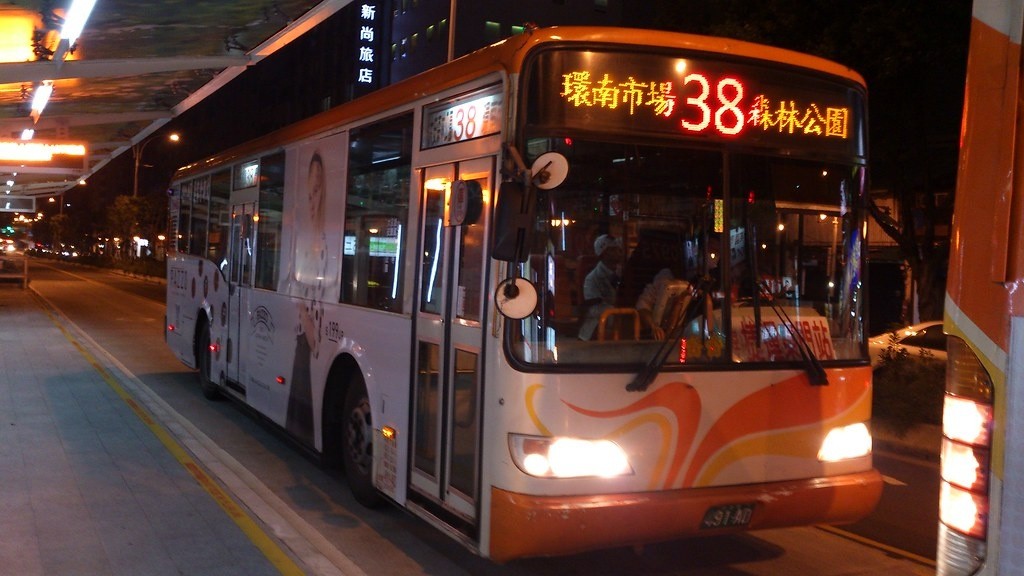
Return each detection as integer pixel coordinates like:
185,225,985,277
594,235,622,256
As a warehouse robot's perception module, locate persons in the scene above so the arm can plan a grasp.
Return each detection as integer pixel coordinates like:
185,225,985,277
285,149,329,449
579,234,722,341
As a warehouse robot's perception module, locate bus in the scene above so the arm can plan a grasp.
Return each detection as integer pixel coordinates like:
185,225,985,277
164,25,888,566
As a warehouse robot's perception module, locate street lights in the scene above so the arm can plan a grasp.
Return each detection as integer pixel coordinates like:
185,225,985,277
132,133,178,196
